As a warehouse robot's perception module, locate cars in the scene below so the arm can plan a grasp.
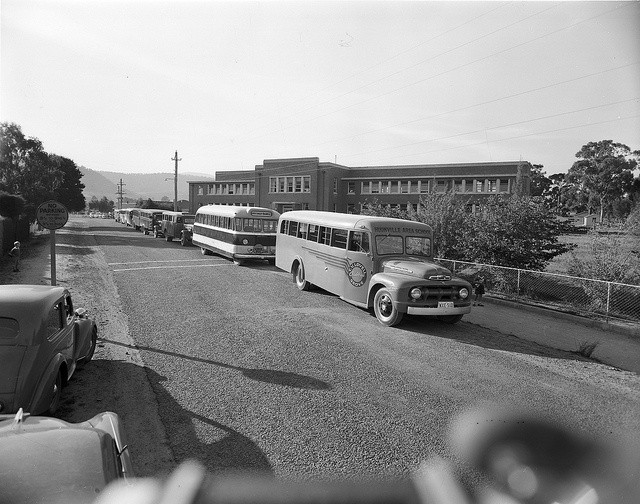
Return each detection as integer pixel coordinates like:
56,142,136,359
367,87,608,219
0,410,134,504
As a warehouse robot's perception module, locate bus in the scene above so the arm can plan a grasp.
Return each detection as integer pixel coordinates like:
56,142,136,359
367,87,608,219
113,209,129,225
134,207,160,238
197,205,282,266
276,209,473,328
162,210,196,246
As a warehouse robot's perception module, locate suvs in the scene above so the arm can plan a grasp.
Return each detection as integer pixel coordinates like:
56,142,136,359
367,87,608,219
1,283,95,418
